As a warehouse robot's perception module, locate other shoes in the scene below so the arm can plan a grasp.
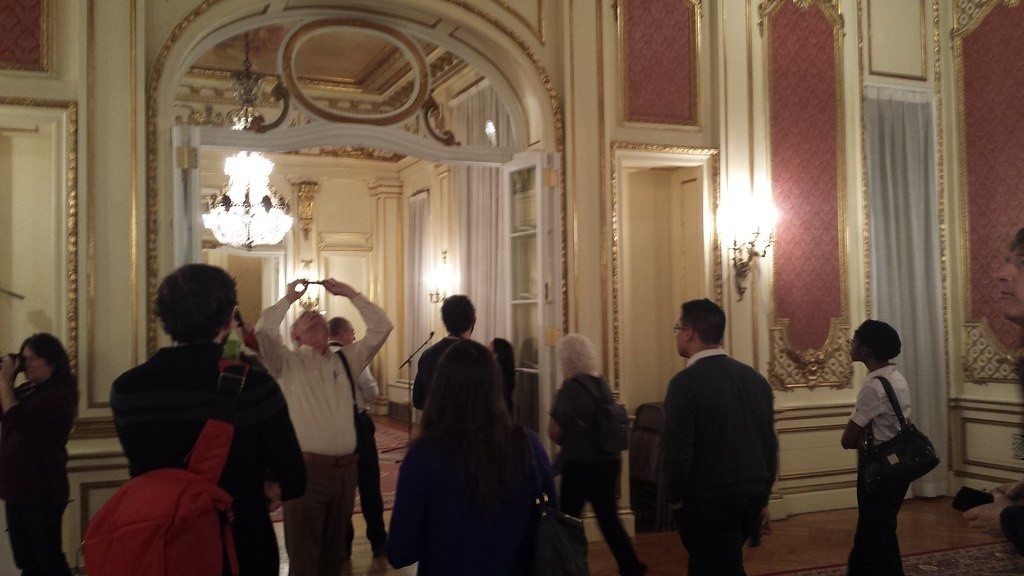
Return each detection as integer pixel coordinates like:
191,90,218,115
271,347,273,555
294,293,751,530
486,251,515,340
373,535,390,554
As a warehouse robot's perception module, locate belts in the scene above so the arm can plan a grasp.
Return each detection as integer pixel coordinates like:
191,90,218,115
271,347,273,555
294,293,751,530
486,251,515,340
303,451,357,465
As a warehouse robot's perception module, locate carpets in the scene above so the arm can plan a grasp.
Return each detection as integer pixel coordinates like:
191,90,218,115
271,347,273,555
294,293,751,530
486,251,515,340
269,456,401,523
758,540,1024,576
372,418,409,454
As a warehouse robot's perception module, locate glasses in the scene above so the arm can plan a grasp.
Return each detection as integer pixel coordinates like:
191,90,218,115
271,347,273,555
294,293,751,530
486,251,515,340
345,328,356,335
850,339,860,348
672,322,688,333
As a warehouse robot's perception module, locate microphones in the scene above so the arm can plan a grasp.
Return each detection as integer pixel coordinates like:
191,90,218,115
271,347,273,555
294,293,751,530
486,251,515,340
431,332,433,338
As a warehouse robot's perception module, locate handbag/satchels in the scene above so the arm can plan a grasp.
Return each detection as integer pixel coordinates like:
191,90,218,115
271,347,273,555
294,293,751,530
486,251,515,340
79,361,252,576
858,375,940,492
517,424,588,576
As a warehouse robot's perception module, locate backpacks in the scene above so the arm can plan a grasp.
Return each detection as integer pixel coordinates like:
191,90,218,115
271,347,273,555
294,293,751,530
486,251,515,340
570,377,629,453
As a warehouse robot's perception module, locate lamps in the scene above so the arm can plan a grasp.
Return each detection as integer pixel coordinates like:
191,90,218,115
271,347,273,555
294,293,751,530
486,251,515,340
716,189,780,300
202,32,293,253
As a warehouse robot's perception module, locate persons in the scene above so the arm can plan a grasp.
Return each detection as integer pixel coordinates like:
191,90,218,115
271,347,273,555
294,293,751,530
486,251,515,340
0,333,79,576
412,294,477,410
548,334,647,576
487,337,515,416
253,277,394,576
386,339,561,576
658,297,777,576
108,263,307,576
963,228,1024,576
327,316,390,556
840,319,912,576
